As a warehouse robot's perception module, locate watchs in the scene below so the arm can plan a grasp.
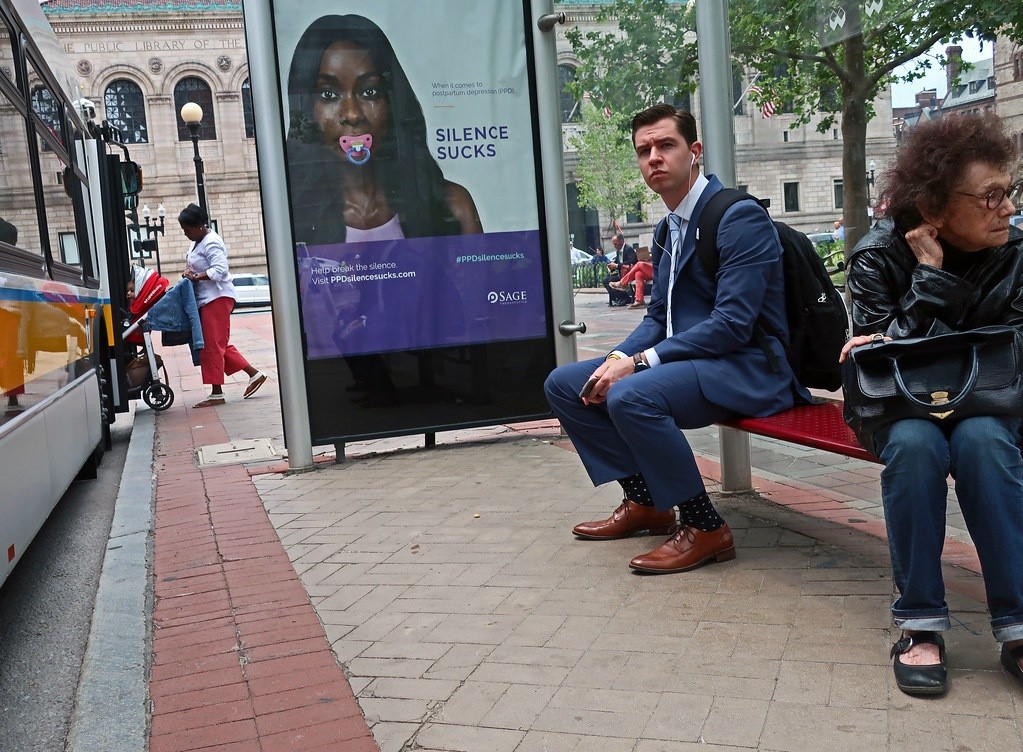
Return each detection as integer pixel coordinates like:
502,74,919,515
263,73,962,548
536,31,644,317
633,353,649,372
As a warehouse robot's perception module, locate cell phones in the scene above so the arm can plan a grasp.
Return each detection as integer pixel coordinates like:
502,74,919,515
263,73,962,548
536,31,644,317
578,378,600,397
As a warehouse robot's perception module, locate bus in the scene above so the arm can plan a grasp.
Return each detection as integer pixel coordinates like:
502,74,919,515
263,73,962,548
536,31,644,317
0,0,144,590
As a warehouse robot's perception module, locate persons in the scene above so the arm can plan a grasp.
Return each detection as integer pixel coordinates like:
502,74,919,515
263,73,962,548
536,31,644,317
0,217,26,413
608,261,653,309
833,217,845,242
544,103,825,573
604,234,638,307
331,243,466,357
839,112,1023,697
591,247,611,265
177,204,268,408
569,240,581,264
127,280,135,303
284,14,484,244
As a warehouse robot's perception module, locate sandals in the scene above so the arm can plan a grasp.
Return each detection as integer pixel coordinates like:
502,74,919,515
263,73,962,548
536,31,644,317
192,392,227,408
244,373,268,399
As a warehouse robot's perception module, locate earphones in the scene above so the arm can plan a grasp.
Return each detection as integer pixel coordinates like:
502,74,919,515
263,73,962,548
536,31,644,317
691,152,695,166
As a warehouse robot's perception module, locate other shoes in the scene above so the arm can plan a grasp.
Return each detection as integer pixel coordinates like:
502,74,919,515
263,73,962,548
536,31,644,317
609,282,628,291
628,301,648,308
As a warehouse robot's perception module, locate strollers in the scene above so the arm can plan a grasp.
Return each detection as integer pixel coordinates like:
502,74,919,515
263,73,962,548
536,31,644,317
119,277,195,411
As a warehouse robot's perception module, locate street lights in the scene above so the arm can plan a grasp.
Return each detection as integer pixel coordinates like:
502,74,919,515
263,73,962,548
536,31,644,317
180,101,210,229
866,159,876,207
141,201,167,278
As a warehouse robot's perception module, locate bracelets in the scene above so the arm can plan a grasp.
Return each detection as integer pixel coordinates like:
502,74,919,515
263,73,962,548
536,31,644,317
193,273,198,280
609,354,621,360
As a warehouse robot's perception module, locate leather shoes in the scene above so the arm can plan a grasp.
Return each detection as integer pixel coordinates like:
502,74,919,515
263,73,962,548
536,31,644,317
889,628,948,694
1000,639,1023,680
629,522,737,573
572,491,678,539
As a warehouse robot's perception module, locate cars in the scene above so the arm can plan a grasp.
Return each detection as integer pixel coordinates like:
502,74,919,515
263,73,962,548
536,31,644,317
229,272,272,307
805,230,837,265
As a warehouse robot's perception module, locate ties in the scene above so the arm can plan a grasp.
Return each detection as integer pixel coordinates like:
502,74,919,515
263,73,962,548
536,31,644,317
666,212,682,273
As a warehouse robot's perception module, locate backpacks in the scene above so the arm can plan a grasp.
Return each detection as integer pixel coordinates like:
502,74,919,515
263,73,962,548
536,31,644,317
657,188,850,392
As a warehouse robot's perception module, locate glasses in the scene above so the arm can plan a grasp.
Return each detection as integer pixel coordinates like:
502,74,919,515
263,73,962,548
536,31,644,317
941,181,1023,210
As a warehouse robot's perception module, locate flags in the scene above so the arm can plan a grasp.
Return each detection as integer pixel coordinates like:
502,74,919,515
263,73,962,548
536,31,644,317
749,73,784,119
582,89,613,120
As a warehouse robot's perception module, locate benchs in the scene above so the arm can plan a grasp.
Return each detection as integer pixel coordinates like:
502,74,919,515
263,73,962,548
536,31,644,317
713,396,881,498
627,279,653,304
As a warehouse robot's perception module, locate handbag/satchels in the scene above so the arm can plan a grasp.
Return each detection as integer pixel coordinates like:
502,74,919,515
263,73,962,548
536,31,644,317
611,296,628,305
125,353,163,387
843,326,1023,455
